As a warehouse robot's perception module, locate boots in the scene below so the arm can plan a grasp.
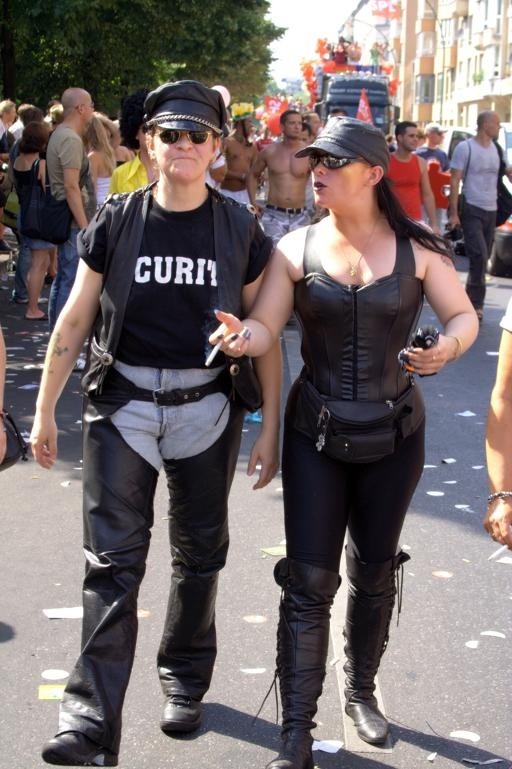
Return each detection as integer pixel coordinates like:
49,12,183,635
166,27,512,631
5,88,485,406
264,552,343,768
339,544,412,745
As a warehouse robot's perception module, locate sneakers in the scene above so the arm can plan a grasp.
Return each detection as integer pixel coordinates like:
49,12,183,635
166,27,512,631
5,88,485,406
71,353,87,371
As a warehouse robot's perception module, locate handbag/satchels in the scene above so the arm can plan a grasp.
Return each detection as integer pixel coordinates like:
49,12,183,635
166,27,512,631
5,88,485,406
19,146,90,246
0,407,29,473
0,183,21,233
296,377,418,464
446,192,467,231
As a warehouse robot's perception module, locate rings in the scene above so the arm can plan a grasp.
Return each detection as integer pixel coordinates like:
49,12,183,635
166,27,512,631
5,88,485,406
488,532,496,539
432,357,436,361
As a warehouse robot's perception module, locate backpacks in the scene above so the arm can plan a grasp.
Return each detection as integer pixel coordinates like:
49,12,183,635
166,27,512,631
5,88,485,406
491,140,512,228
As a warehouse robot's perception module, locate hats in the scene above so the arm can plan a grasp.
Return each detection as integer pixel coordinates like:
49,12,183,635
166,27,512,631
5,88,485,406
424,122,449,136
140,80,227,138
295,115,392,163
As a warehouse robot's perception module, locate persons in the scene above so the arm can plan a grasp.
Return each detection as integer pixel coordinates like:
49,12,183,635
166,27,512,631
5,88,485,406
0,325,9,467
200,111,480,768
28,80,284,769
482,300,512,549
385,112,511,316
1,88,158,370
205,92,348,325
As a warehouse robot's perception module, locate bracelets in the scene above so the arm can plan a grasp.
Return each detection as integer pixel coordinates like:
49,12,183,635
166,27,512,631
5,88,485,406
453,335,462,360
485,491,512,500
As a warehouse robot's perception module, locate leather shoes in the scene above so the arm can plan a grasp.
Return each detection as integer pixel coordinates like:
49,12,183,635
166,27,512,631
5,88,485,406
159,694,204,737
41,732,121,769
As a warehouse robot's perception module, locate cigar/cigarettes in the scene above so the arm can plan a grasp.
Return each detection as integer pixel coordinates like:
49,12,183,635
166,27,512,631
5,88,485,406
204,341,223,367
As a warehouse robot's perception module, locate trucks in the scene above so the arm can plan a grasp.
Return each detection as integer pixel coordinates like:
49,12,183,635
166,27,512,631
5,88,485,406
310,64,400,141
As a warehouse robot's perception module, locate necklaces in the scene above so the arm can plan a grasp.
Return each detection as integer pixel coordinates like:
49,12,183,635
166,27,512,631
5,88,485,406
332,223,381,277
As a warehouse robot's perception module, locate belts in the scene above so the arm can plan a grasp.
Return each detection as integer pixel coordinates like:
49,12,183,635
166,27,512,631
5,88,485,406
265,204,309,214
105,380,226,407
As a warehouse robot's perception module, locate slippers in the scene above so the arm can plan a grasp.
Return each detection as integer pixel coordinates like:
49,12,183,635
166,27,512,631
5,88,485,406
23,311,49,321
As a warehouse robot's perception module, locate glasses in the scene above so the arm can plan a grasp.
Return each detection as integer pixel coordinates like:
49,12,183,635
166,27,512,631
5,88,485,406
75,102,95,110
146,126,219,146
308,152,371,170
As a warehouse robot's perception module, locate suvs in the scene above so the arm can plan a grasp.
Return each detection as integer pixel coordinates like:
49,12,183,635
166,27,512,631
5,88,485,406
430,124,512,277
